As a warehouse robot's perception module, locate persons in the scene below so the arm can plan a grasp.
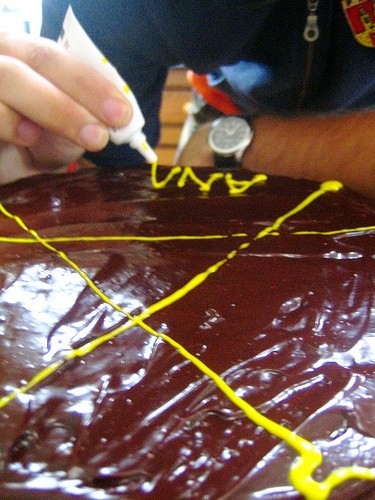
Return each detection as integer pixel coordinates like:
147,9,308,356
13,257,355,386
0,0,375,201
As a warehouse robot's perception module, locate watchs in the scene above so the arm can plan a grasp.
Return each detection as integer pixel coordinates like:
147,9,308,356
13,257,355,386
208,114,255,168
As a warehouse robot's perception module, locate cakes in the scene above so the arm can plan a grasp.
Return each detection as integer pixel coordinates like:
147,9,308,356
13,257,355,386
0,165,375,500
